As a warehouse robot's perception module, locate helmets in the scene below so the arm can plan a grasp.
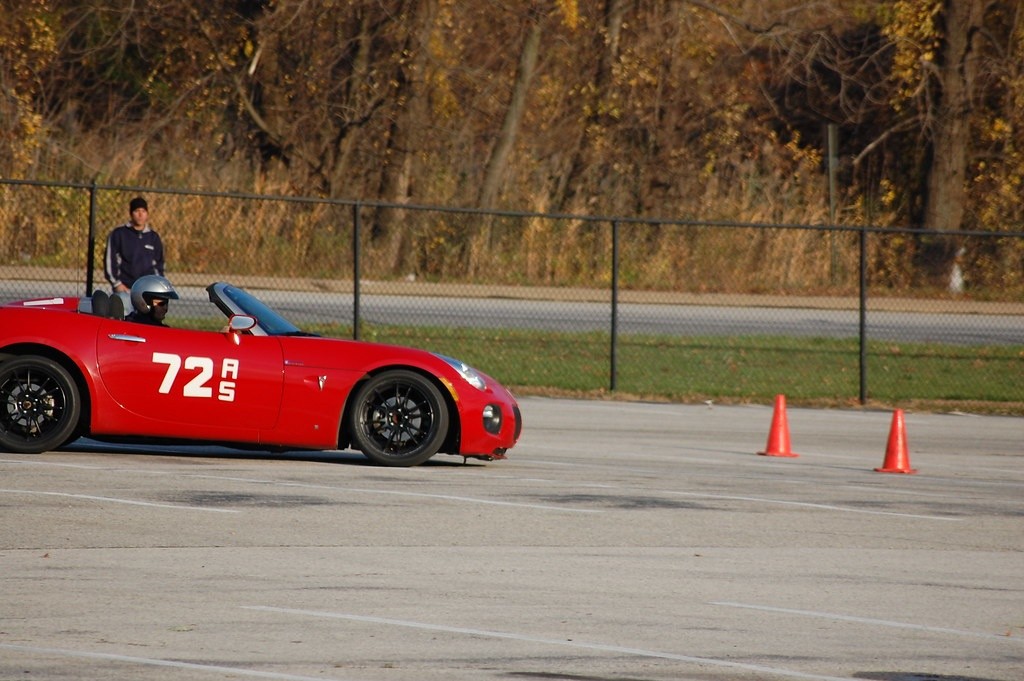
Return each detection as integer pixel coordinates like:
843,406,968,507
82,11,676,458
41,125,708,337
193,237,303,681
130,275,179,314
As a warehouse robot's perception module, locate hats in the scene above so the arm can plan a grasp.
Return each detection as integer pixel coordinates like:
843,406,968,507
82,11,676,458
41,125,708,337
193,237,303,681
130,198,147,214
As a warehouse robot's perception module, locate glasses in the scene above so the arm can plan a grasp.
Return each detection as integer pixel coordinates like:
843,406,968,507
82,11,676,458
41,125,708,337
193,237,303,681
153,300,168,306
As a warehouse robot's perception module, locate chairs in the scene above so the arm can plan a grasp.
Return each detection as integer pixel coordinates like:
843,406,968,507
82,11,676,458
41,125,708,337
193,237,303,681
109,294,124,320
91,290,110,316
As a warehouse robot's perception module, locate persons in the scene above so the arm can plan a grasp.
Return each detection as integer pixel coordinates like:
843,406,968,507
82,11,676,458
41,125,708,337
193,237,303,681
103,196,166,317
124,275,179,327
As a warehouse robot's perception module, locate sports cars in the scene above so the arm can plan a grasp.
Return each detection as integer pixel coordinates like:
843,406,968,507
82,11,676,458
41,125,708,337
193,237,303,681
0,278,524,468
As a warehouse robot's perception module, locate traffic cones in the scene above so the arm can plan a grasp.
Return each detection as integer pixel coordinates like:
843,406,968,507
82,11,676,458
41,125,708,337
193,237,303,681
758,393,799,458
874,405,918,476
